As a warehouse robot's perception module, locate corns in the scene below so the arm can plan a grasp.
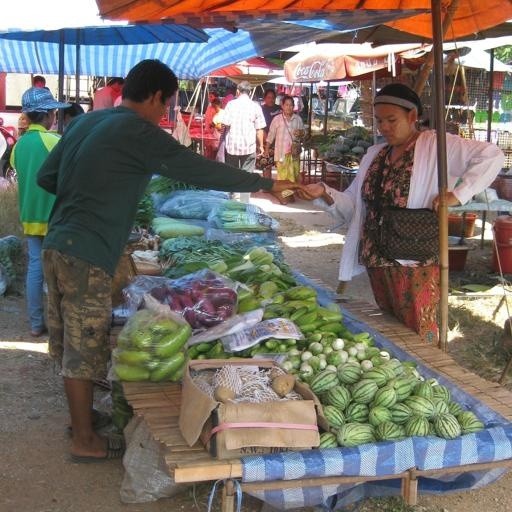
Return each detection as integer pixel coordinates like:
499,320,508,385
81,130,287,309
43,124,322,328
219,201,273,232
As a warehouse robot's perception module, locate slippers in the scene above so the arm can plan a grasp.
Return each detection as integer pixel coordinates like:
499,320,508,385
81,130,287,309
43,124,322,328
63,409,127,460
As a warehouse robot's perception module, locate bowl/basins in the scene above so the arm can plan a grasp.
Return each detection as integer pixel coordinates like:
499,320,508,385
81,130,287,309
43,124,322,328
448,247,469,269
448,213,477,237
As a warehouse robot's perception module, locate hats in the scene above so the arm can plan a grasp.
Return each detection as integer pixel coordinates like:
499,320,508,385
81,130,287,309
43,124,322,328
20,87,73,113
372,82,423,117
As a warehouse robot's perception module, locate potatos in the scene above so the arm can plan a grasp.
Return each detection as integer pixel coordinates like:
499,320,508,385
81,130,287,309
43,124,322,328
191,366,303,404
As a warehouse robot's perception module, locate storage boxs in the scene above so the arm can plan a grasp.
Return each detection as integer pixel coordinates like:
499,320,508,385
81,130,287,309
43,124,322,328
182,357,324,458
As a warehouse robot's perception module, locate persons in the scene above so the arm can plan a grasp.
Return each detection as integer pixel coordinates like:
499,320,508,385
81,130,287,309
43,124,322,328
295,83,505,345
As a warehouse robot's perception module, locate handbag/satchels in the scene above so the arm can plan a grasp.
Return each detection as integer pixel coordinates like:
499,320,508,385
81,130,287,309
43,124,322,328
291,140,305,157
376,204,441,262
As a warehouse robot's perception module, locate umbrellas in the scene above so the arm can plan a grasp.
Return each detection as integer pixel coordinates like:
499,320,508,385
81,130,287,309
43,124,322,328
89,1,512,351
0,8,435,137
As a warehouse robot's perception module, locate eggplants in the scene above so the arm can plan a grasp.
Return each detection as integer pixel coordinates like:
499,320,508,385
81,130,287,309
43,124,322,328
333,138,372,155
112,245,370,381
152,216,204,237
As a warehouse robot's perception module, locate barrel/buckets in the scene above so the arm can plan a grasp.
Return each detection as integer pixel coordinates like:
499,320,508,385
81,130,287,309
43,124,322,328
492,214,512,274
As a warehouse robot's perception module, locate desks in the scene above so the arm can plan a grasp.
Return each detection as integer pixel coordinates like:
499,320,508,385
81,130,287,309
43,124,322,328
445,196,512,249
108,323,512,511
295,139,358,191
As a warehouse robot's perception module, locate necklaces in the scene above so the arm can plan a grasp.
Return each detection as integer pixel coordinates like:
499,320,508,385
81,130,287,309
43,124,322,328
378,147,404,189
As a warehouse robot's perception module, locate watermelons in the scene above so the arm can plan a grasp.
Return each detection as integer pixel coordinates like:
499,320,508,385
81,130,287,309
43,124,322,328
310,359,484,449
